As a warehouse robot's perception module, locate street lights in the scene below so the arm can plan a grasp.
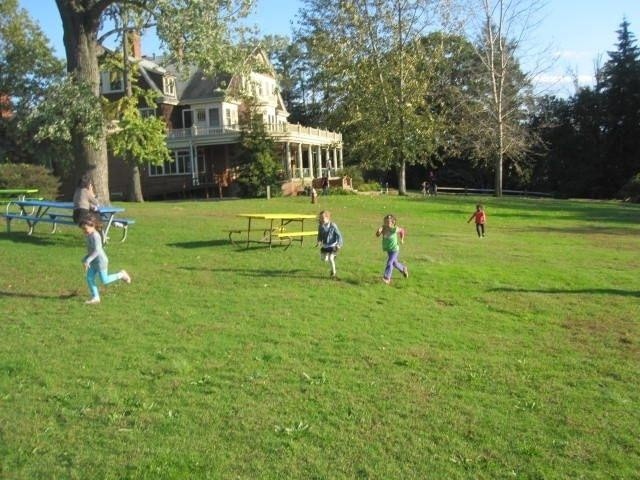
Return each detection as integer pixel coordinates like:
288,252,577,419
190,124,200,186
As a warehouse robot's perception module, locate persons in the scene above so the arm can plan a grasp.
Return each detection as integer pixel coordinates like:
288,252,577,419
314,210,343,276
73,174,109,247
420,180,437,197
467,205,487,238
376,214,408,284
321,173,331,196
77,214,131,304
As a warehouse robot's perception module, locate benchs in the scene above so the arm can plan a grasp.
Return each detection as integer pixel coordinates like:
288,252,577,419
274,230,321,251
220,228,285,251
0,186,137,245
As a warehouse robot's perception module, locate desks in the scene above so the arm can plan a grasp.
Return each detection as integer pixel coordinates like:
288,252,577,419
233,213,319,251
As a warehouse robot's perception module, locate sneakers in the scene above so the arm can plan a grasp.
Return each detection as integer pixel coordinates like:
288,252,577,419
120,269,132,284
84,298,101,304
402,265,409,279
